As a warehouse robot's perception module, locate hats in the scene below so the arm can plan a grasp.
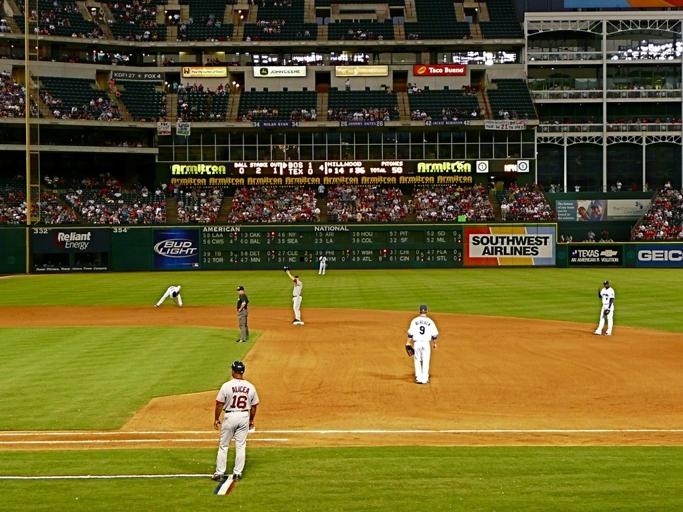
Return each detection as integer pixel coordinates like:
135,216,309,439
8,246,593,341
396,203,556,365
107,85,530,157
231,361,245,375
603,280,610,284
419,305,428,314
236,286,244,291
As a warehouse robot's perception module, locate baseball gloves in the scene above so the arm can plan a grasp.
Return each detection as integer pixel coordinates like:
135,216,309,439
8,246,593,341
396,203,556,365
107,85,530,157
603,309,611,316
283,265,288,271
404,345,414,357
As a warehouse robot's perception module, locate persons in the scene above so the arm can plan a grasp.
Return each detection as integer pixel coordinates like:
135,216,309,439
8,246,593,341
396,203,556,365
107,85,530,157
404,303,440,385
592,280,616,335
210,361,259,480
68,134,147,147
282,266,305,325
233,284,248,342
553,38,681,143
315,254,328,275
151,285,182,308
0,0,529,122
553,174,682,244
1,174,552,226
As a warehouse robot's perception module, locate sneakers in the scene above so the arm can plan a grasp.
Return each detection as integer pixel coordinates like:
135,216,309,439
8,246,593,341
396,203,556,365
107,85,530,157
236,337,249,343
292,319,302,324
232,473,242,481
211,474,225,483
